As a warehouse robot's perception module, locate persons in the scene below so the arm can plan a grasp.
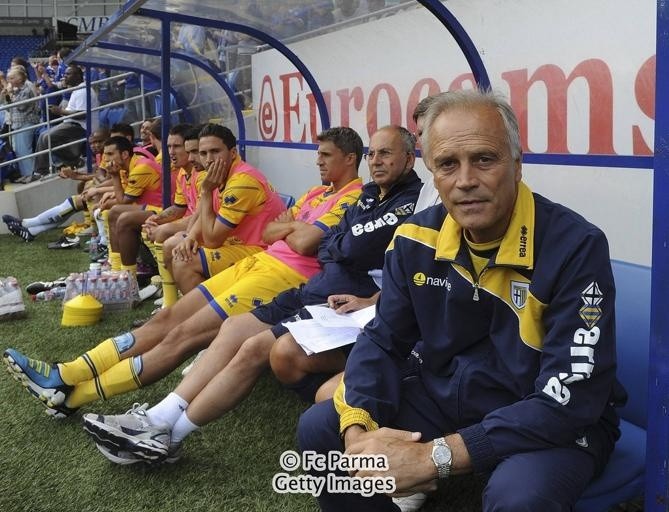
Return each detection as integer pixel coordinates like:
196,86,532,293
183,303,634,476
0,46,106,189
2,87,444,471
105,1,251,119
251,1,416,50
284,83,631,511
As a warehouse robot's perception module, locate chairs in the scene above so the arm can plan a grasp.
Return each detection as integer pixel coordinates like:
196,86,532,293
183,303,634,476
473,257,652,508
86,67,237,132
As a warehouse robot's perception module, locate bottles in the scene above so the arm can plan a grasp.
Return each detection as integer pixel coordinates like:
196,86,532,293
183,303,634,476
89,233,98,260
0,275,22,298
31,287,66,302
63,259,142,304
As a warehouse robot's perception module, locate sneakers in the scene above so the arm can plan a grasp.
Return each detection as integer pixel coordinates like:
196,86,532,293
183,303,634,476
150,290,185,315
81,411,175,462
93,438,186,467
14,176,37,183
47,237,80,251
2,214,35,243
26,276,67,294
2,347,79,419
74,227,108,263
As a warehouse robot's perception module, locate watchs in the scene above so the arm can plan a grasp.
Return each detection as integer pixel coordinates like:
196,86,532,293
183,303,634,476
432,436,452,479
